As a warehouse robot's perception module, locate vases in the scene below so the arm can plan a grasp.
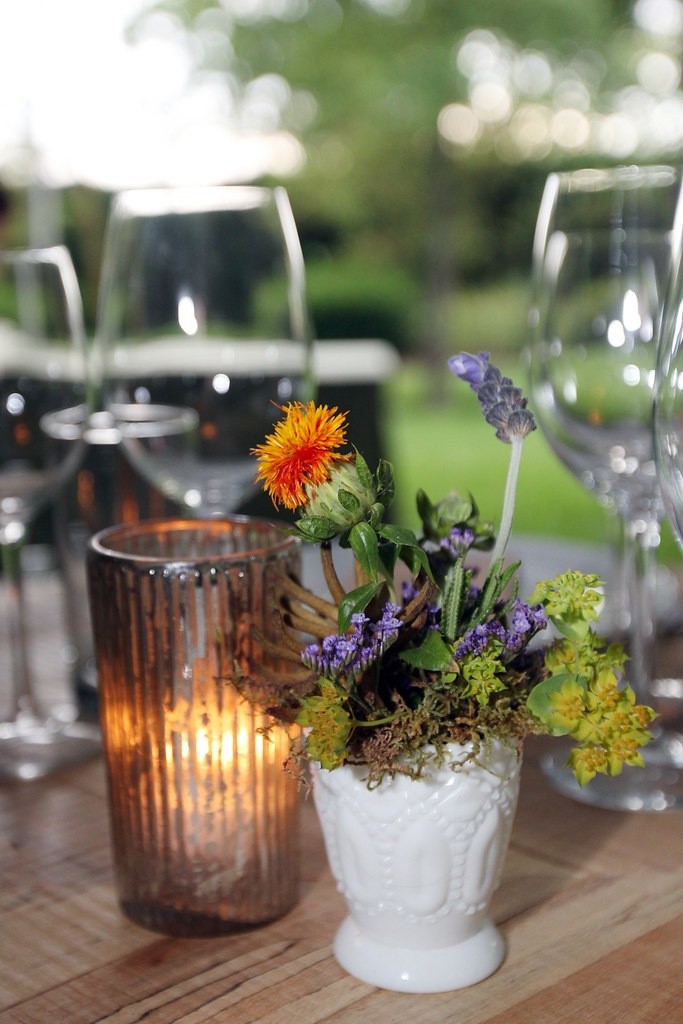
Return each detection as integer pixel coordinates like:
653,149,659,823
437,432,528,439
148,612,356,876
306,734,525,996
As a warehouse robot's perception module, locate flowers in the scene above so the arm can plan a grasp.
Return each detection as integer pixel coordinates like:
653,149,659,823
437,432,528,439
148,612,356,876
229,348,659,789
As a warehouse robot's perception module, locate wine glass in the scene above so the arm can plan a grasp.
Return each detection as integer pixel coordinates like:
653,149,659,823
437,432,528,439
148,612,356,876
543,233,683,721
530,166,683,812
0,246,101,777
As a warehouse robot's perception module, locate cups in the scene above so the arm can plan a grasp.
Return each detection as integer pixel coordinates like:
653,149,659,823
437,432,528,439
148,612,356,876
42,402,201,723
652,234,683,550
95,184,314,518
86,518,305,936
